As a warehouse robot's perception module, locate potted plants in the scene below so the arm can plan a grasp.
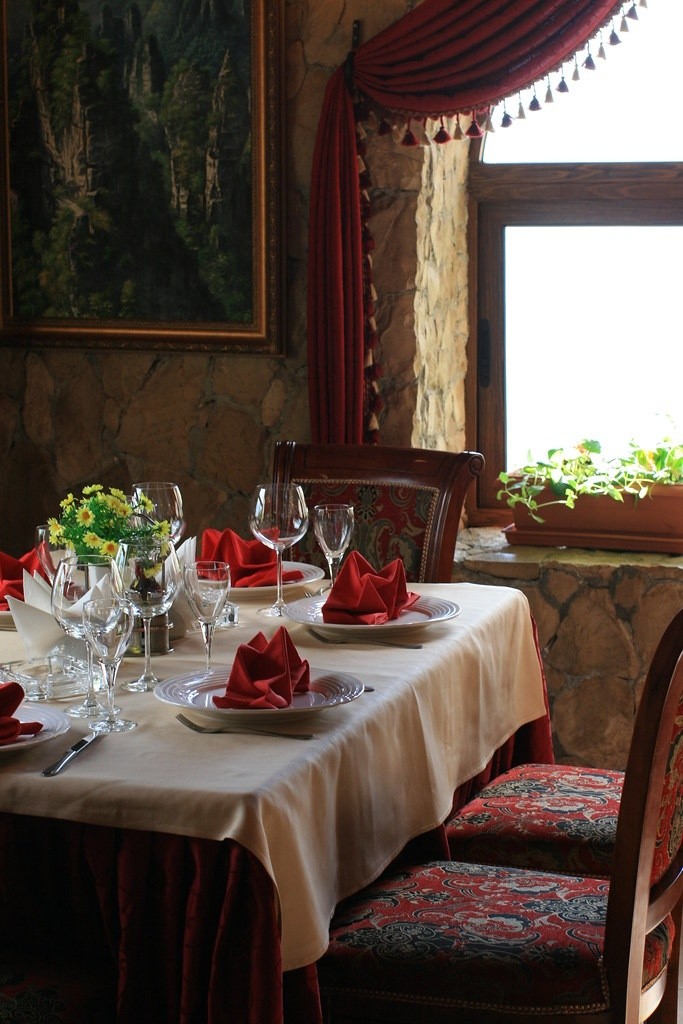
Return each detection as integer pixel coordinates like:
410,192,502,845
496,435,683,553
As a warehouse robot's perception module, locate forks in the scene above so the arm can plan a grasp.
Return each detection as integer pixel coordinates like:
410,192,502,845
305,626,423,650
174,713,314,740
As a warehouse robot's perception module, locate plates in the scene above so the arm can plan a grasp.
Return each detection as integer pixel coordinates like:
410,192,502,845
153,667,365,715
230,560,325,595
0,704,72,752
284,593,461,629
0,611,18,632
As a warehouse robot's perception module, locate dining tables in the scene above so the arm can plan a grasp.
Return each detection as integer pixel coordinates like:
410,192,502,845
0,579,554,1024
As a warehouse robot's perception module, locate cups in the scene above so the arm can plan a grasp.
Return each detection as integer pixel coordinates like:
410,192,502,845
131,482,185,545
36,525,78,587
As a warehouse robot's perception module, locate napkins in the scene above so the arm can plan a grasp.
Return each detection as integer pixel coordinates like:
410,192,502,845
6,537,201,657
0,682,42,747
212,626,310,710
196,529,302,587
321,552,420,623
0,542,74,611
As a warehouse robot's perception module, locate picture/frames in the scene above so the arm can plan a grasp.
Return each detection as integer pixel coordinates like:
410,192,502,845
0,0,286,357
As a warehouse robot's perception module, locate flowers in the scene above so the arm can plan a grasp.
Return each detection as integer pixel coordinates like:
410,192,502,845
47,485,170,577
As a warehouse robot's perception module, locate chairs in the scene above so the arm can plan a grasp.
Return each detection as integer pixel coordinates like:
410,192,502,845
322,608,683,1024
272,441,485,582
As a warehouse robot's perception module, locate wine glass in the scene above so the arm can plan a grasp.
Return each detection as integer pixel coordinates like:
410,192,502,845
51,555,124,718
183,561,231,668
314,503,354,586
82,598,139,733
115,538,181,693
249,483,309,618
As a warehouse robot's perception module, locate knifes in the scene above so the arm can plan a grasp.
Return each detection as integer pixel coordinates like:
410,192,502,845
40,733,99,777
